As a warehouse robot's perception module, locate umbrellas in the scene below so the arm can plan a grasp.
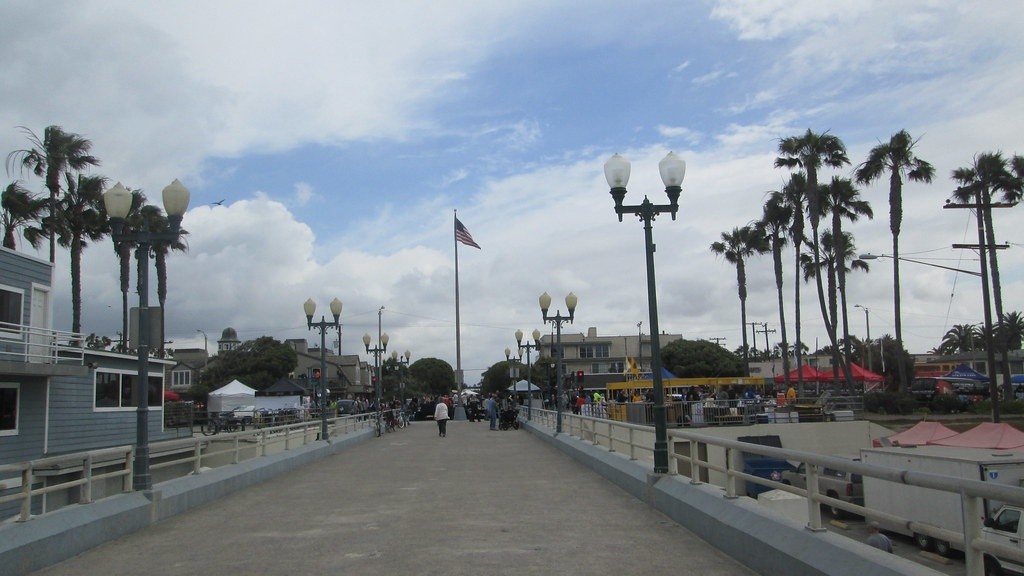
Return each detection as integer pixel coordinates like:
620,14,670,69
505,378,541,391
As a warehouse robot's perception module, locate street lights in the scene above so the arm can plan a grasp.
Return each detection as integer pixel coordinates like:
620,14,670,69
605,148,689,472
505,346,524,405
303,296,343,439
860,252,1001,424
363,335,389,436
196,329,208,367
391,350,412,428
104,174,192,486
515,328,541,420
539,290,577,434
854,304,872,372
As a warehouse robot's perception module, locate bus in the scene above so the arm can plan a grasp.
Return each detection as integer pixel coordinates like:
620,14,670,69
912,377,977,399
913,361,963,377
568,388,608,403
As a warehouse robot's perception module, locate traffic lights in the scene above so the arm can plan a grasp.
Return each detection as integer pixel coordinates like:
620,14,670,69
577,371,585,383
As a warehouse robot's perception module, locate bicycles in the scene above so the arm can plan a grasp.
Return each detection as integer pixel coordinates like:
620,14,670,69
258,406,300,425
201,406,245,437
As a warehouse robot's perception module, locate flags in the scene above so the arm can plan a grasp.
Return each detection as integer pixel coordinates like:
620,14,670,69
455,217,481,249
630,355,639,374
624,356,631,376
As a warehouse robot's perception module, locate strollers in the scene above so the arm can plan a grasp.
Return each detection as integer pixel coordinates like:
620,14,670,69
498,408,519,431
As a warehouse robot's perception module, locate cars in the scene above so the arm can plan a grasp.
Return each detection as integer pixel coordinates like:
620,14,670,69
337,400,359,414
780,455,864,519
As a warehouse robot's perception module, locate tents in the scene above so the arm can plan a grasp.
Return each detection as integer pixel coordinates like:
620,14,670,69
208,379,258,423
816,361,884,397
254,374,307,421
940,362,991,393
772,364,826,395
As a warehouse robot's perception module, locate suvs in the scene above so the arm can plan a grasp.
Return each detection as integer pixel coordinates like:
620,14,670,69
996,382,1024,403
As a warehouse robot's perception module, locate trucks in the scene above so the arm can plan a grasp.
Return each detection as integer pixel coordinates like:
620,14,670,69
861,443,1024,576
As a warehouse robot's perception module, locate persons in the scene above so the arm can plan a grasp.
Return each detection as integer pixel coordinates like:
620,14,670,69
686,386,700,415
434,397,450,437
616,389,655,406
355,396,402,422
550,393,557,411
563,390,569,410
445,394,459,408
786,383,796,404
571,392,607,415
865,522,892,554
462,393,514,431
